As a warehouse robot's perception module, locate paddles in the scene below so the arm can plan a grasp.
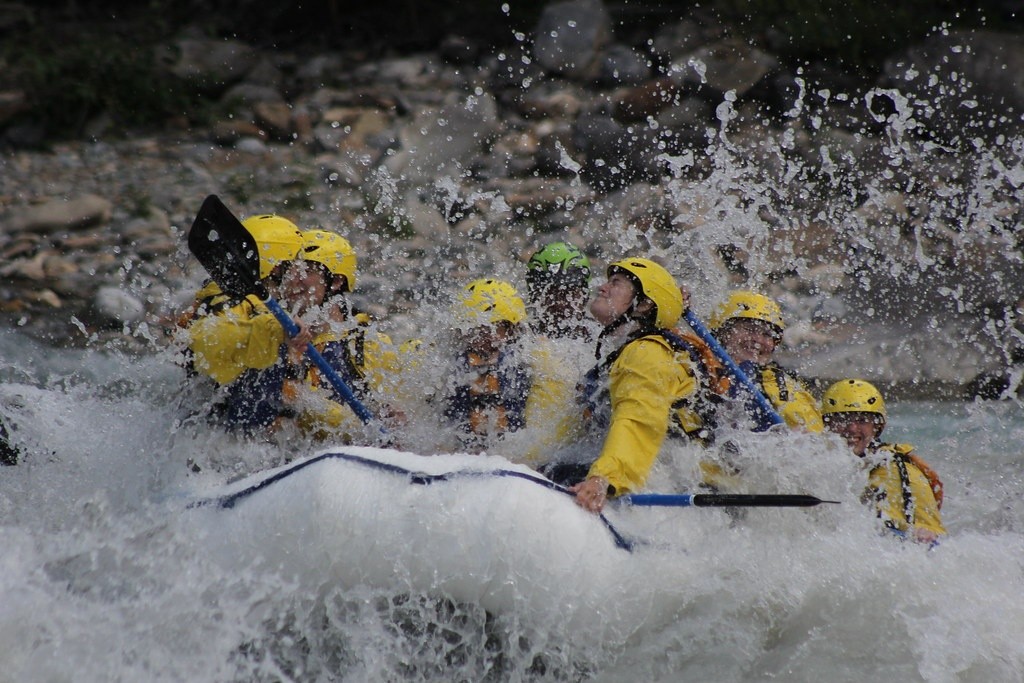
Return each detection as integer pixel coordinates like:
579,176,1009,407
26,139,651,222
681,305,788,433
615,489,845,510
186,192,398,447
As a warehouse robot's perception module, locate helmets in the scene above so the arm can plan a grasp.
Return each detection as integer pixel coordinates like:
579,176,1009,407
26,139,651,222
526,240,591,287
708,291,783,346
242,214,305,279
607,257,682,328
298,228,356,294
822,378,887,436
456,277,525,324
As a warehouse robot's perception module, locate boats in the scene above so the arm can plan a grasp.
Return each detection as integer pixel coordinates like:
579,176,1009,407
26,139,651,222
184,445,634,624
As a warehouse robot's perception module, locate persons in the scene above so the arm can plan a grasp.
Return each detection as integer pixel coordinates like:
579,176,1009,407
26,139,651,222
181,214,944,548
